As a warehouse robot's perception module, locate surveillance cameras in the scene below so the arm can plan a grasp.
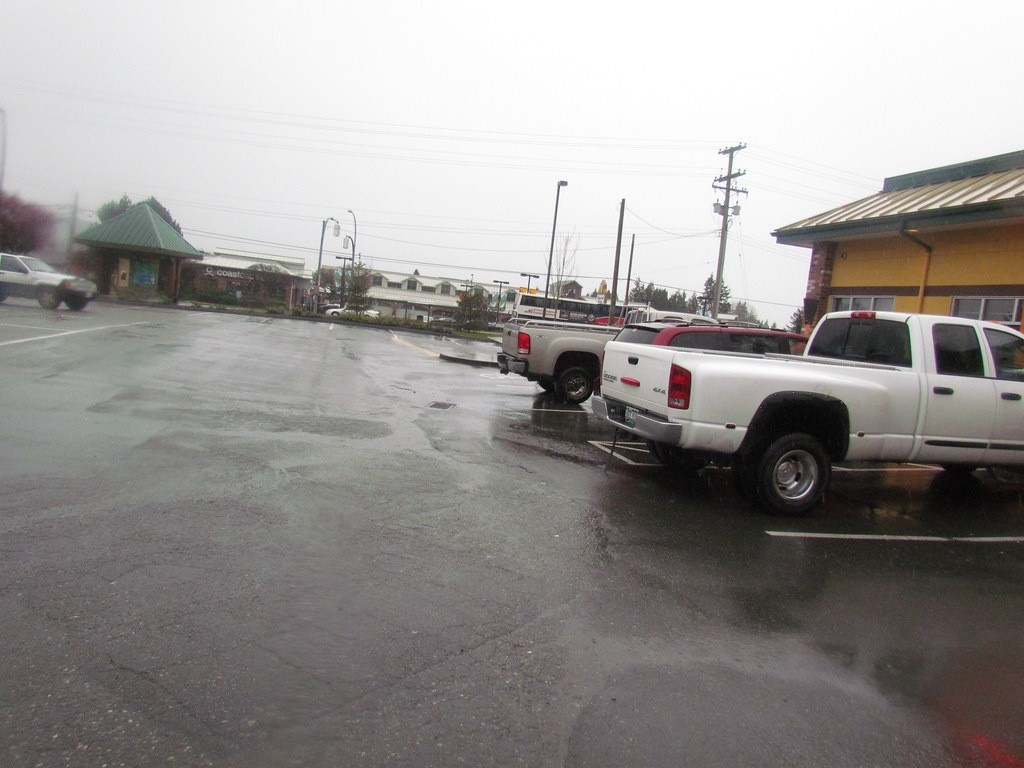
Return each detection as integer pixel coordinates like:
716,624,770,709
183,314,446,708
840,253,846,259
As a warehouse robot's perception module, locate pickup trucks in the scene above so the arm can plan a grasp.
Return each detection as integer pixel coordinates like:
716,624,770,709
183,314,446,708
496,302,720,406
590,306,1024,518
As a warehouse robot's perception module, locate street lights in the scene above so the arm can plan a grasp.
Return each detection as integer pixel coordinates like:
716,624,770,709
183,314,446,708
342,210,357,267
520,273,540,294
336,256,352,308
467,285,476,300
461,283,470,333
542,180,568,318
493,279,509,323
316,217,342,301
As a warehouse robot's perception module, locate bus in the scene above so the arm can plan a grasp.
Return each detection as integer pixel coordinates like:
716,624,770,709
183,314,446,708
510,292,658,326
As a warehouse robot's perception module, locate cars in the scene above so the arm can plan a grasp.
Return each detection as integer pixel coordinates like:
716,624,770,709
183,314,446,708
0,252,98,311
430,317,459,328
318,301,379,320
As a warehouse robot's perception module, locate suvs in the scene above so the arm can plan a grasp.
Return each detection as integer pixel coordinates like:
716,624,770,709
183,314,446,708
611,321,822,358
